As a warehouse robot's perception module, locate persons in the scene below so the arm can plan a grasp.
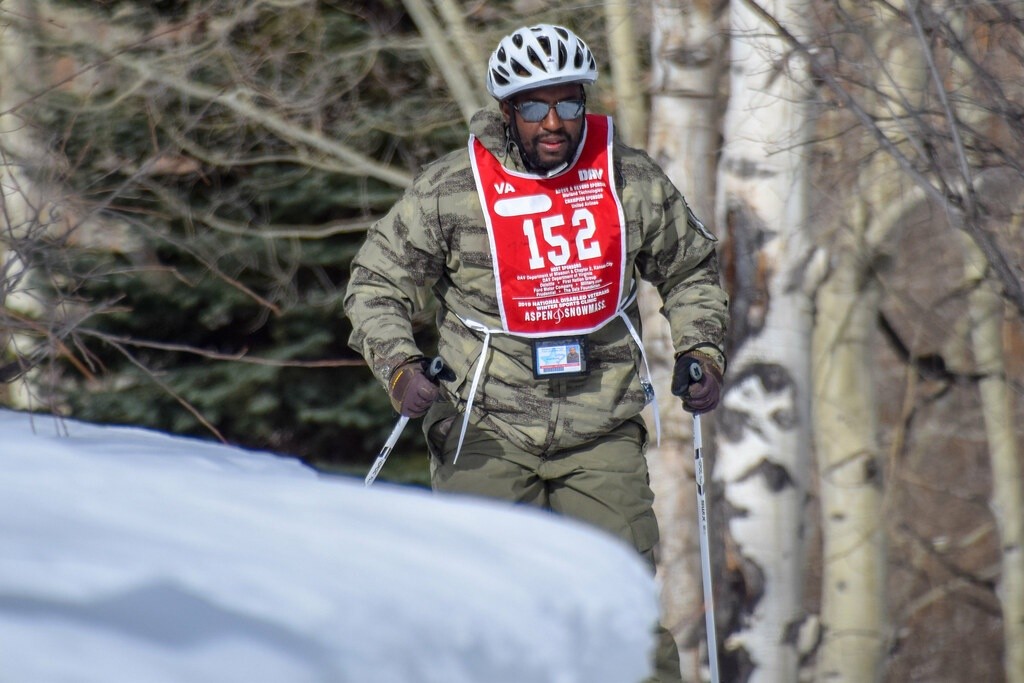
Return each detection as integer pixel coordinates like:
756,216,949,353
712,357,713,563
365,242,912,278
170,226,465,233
568,346,580,363
345,23,731,683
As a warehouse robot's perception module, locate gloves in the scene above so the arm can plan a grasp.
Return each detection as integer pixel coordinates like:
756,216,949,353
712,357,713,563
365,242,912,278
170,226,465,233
386,356,457,419
671,341,727,414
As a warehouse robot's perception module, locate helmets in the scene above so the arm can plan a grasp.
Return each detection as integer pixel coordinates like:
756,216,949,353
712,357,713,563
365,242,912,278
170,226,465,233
486,24,600,101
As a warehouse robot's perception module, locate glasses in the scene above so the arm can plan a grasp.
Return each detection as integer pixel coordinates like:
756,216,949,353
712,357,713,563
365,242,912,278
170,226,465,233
508,97,587,123
